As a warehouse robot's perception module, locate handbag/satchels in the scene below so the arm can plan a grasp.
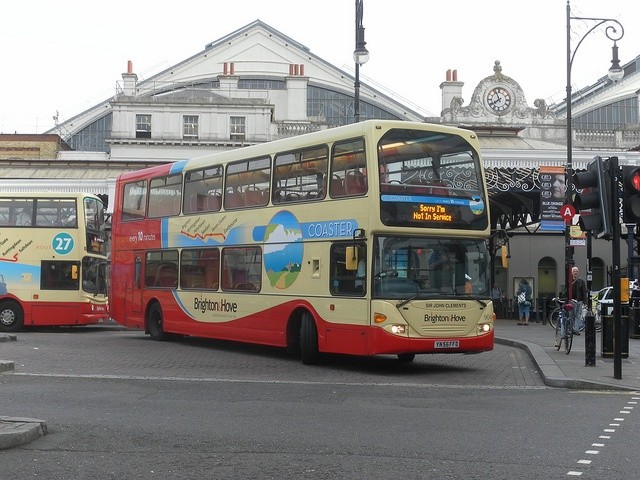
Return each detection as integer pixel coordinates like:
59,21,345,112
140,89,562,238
517,285,526,304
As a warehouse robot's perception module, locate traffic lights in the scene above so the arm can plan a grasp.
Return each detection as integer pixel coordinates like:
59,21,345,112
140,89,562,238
571,157,612,240
620,163,640,225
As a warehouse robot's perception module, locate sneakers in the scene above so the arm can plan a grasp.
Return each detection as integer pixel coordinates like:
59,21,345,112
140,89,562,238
576,331,580,335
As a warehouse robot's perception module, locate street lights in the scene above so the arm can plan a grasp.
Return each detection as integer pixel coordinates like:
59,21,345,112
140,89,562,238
564,0,624,298
352,0,369,123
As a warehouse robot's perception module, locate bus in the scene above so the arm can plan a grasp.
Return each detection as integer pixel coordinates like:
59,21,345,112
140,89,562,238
0,192,109,333
108,119,511,365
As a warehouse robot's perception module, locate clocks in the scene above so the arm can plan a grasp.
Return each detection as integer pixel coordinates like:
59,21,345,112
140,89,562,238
483,85,514,114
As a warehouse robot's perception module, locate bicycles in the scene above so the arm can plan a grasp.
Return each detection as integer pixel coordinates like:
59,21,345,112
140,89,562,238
550,297,574,354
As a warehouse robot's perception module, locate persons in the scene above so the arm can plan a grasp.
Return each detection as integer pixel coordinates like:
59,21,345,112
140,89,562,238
492,282,503,321
553,174,567,201
516,277,533,326
569,265,588,337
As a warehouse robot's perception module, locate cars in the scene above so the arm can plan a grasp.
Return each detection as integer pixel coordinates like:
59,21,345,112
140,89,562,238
595,287,616,316
